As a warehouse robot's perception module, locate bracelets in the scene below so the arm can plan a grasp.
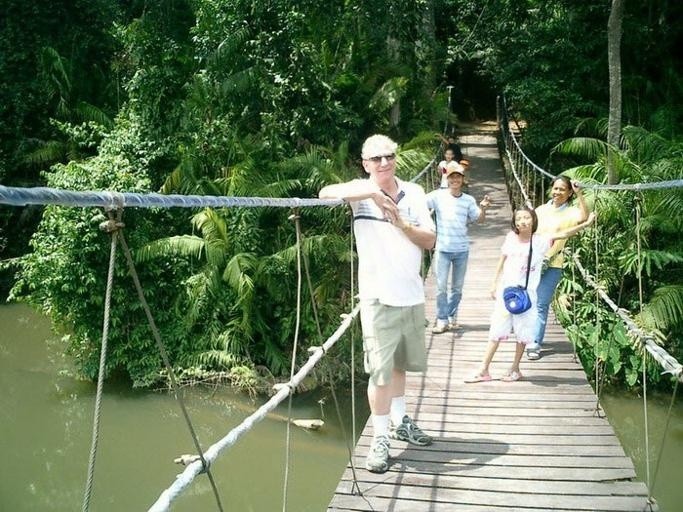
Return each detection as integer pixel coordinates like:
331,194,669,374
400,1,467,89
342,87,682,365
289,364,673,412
582,221,586,230
401,221,412,233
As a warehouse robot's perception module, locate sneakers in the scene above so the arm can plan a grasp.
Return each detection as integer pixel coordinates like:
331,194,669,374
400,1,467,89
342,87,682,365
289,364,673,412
366,434,390,473
389,415,433,447
433,319,462,333
464,374,491,382
526,348,539,359
502,372,521,381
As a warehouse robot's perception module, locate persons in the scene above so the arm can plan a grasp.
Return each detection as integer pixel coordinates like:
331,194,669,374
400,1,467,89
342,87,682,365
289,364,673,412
462,201,599,386
421,163,492,335
524,175,589,362
457,159,473,193
436,148,459,190
316,133,438,473
433,133,463,163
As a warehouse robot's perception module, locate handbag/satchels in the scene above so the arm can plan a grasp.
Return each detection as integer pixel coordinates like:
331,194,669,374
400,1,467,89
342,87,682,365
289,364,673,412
541,256,550,275
503,286,531,314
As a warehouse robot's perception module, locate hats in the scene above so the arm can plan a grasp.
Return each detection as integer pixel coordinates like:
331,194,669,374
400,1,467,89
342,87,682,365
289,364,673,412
445,164,466,179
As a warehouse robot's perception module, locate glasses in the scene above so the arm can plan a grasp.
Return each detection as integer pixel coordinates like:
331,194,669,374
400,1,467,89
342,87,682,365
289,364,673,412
363,153,395,162
